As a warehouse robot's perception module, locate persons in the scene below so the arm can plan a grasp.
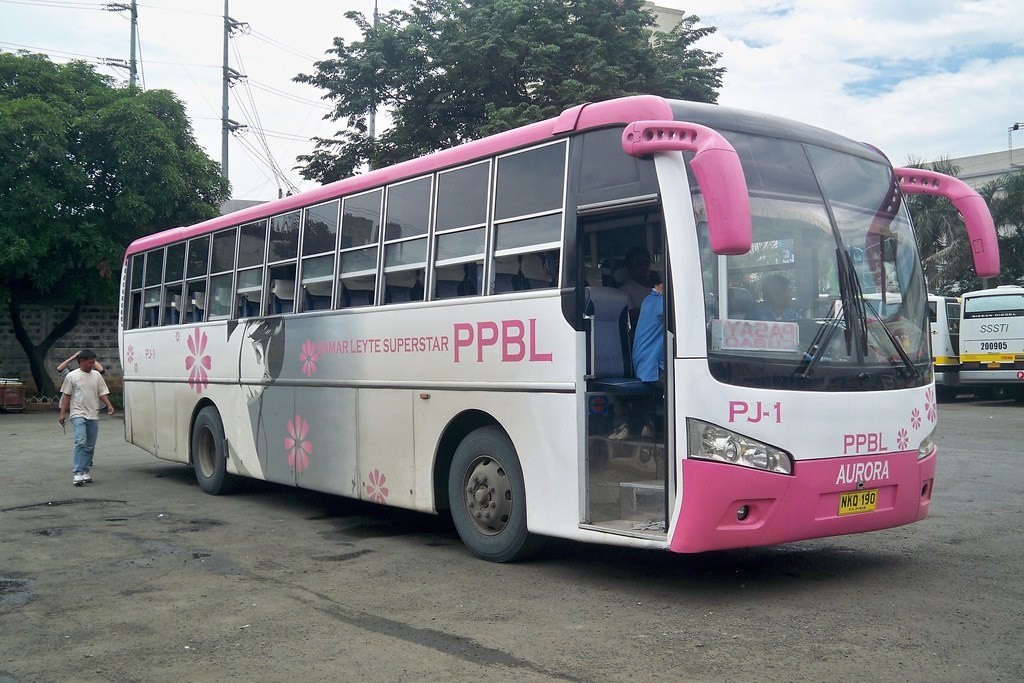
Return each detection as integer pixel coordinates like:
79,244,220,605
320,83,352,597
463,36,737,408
57,351,108,466
633,268,667,389
604,395,659,440
744,275,804,321
59,349,114,486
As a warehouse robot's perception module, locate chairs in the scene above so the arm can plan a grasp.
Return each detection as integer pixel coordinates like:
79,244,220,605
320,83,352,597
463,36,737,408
148,252,630,327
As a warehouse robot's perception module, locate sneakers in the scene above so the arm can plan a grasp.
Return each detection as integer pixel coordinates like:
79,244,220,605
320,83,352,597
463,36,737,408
642,426,659,441
73,474,83,485
82,473,93,482
609,426,632,440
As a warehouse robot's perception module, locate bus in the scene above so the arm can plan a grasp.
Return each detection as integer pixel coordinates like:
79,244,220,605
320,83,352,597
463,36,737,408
810,284,1024,402
119,94,1002,563
